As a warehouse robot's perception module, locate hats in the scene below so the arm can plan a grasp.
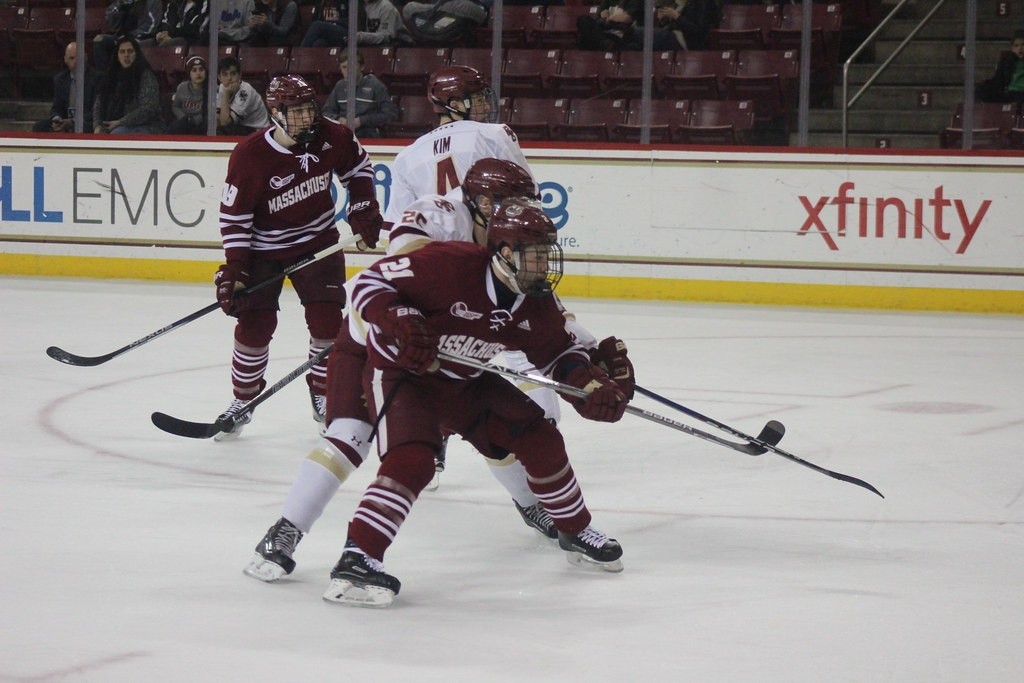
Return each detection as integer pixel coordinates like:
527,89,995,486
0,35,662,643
184,55,208,79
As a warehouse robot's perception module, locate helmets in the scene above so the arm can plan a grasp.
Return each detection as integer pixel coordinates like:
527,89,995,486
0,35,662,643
428,65,497,124
266,74,321,144
461,156,537,228
487,200,564,299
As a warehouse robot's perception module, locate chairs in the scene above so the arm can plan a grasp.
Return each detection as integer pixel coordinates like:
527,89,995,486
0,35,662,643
943,51,1024,149
0,0,876,146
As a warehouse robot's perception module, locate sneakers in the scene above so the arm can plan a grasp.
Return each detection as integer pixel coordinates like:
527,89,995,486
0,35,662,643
306,374,330,437
558,525,623,573
213,378,267,442
512,498,560,546
323,522,401,608
243,517,304,583
424,438,448,491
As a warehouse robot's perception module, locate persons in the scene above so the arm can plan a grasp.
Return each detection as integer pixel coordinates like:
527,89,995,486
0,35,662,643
974,29,1024,115
328,194,623,595
139,0,209,46
92,35,167,135
342,0,416,47
575,0,722,52
249,0,300,46
217,0,255,45
321,48,392,137
153,53,208,134
214,72,385,431
217,54,270,134
254,154,596,573
93,0,163,45
378,63,560,428
301,0,367,47
31,41,104,134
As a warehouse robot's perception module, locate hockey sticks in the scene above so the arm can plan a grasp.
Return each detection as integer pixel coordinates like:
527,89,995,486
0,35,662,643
47,233,363,368
634,384,886,502
150,344,331,439
436,351,786,457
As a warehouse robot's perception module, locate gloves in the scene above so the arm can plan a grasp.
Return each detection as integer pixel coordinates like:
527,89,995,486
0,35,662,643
555,356,628,422
364,292,439,374
586,336,636,400
215,261,250,318
347,200,383,251
